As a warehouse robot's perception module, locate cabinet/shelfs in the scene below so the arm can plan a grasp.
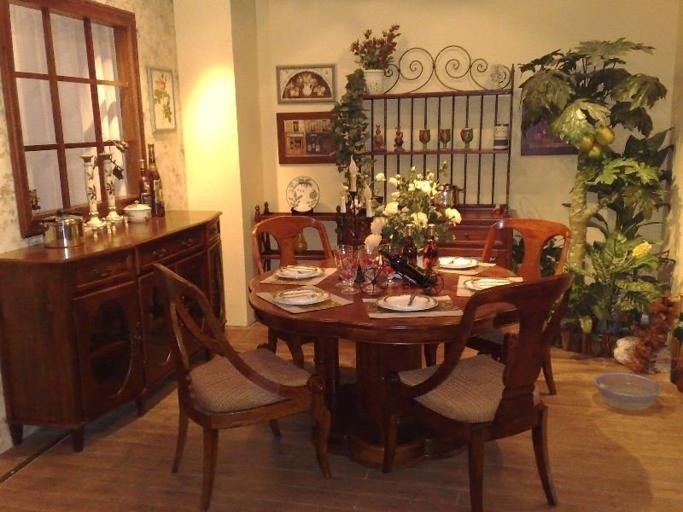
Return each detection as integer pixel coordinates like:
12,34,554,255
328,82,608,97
0,208,225,453
338,211,512,269
254,200,345,273
355,88,515,209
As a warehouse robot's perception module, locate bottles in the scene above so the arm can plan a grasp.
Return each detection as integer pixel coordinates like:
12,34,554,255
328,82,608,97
147,142,166,217
402,223,417,268
378,249,432,289
138,158,151,206
424,223,439,274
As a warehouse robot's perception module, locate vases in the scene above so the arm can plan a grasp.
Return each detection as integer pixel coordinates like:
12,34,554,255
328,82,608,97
363,69,385,95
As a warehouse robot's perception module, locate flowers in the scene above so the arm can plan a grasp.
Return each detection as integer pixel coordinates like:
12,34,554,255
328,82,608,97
351,160,462,257
349,24,402,69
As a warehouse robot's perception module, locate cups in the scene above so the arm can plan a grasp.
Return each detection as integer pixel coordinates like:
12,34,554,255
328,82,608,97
493,123,509,151
122,200,152,223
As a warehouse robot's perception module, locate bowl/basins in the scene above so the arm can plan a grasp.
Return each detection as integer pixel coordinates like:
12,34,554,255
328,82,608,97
594,371,662,413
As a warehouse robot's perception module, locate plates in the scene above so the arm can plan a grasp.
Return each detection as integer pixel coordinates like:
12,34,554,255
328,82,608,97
272,289,331,306
437,255,480,269
463,278,515,290
285,176,320,215
375,294,438,313
275,263,324,279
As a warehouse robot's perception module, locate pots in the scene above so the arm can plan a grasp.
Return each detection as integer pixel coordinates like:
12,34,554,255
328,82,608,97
36,209,84,248
434,184,465,212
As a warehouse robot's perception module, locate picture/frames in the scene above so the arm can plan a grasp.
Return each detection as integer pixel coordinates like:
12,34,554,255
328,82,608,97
274,63,336,104
517,114,579,157
144,64,178,134
275,111,339,166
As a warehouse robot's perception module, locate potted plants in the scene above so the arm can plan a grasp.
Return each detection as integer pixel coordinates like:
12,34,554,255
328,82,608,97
518,36,671,357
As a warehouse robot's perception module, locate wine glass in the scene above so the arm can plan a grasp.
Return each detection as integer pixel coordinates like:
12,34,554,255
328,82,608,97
334,242,400,293
460,126,473,150
418,129,431,153
438,127,451,150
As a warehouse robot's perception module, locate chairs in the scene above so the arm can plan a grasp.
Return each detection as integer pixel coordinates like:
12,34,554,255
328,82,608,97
443,217,570,395
152,260,334,511
248,215,337,368
380,271,580,511
